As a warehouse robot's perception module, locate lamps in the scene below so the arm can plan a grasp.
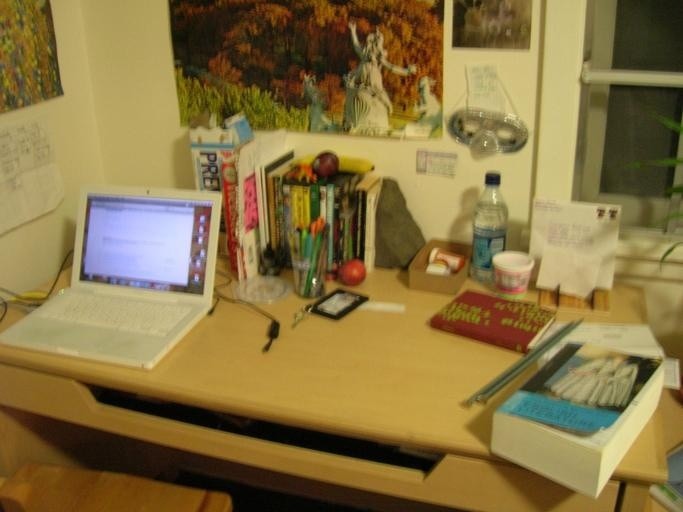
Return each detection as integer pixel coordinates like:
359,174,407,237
446,108,530,164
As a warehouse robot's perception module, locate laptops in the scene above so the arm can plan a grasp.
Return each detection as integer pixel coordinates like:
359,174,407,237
0,186,224,372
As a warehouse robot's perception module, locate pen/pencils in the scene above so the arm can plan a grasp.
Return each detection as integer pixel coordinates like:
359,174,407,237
0,291,47,303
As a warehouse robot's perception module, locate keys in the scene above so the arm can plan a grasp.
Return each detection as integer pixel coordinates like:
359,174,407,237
291,303,313,328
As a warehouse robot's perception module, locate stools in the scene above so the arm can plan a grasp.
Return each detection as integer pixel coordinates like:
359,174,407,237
0,461,233,511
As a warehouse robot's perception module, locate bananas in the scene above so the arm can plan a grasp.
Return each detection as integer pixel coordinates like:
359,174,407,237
292,156,376,175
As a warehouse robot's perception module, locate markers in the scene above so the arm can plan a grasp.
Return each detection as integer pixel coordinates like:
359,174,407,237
288,217,330,298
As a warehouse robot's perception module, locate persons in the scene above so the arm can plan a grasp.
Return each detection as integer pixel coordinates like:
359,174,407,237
303,21,442,140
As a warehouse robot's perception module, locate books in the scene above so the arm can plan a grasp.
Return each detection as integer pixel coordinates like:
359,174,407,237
490,342,665,500
428,289,557,355
219,127,384,281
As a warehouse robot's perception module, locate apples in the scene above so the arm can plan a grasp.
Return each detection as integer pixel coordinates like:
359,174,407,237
312,151,339,177
337,258,366,286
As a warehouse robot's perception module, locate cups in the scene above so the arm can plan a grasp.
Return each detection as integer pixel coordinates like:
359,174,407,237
492,250,533,300
287,228,329,299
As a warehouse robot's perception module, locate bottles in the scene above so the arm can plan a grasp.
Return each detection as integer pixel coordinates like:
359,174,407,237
469,172,509,283
428,248,464,273
425,258,449,276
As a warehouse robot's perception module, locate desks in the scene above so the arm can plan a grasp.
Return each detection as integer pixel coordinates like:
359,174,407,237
0,266,683,512
